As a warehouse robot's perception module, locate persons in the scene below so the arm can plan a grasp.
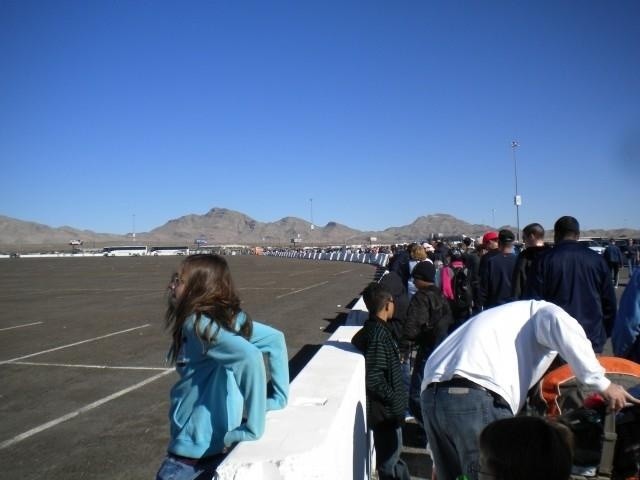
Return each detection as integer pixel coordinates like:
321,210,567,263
263,245,391,256
154,253,290,480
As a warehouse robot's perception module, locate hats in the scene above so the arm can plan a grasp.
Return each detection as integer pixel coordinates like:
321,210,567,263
499,229,514,243
483,231,498,243
473,238,484,246
412,261,435,283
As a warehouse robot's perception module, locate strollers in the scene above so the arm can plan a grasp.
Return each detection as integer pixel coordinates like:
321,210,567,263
529,356,639,480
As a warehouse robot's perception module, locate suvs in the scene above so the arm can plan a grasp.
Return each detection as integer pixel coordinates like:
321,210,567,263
576,238,606,255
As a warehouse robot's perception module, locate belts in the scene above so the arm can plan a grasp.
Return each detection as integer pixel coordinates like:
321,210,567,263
428,377,489,393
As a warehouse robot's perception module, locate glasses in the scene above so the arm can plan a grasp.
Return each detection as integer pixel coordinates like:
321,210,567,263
467,460,496,477
172,273,184,284
390,300,396,304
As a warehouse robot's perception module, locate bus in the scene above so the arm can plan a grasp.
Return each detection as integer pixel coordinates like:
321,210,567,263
102,246,146,257
150,247,187,256
